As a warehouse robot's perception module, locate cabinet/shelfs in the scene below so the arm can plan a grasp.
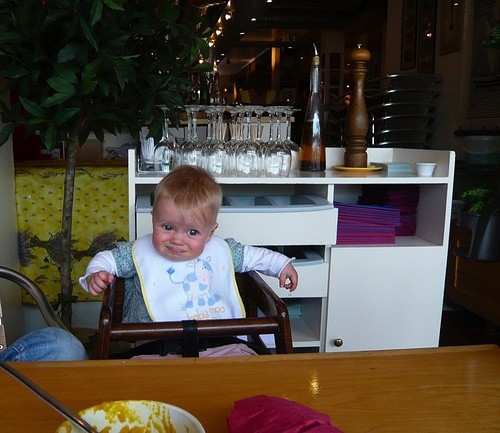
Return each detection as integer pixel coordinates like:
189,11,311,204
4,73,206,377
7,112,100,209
127,145,457,352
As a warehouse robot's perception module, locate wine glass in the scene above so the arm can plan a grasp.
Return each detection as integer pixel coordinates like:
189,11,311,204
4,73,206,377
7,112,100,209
153,104,301,177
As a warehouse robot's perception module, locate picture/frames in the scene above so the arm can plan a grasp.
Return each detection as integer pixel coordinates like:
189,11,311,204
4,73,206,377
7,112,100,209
400,0,463,74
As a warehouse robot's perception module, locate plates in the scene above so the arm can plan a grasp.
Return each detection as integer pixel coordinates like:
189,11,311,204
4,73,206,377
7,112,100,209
333,164,383,172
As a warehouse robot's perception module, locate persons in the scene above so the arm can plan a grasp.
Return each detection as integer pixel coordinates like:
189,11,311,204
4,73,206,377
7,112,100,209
0,327,85,361
78,165,298,360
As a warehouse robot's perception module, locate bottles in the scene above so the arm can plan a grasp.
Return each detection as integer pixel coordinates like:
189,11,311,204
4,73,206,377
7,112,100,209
298,43,327,172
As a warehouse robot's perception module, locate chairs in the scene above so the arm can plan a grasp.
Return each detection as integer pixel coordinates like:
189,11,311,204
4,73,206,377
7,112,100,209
328,72,440,149
0,266,70,331
95,267,296,359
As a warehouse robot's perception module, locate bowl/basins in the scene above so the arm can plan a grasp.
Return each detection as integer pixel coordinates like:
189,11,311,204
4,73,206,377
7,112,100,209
416,162,437,177
57,399,206,433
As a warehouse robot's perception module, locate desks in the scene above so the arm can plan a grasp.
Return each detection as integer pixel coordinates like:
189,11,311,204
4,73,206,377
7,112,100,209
0,343,500,433
446,220,500,330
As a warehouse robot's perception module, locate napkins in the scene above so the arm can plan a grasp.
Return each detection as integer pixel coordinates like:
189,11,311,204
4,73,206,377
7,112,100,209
228,395,346,433
